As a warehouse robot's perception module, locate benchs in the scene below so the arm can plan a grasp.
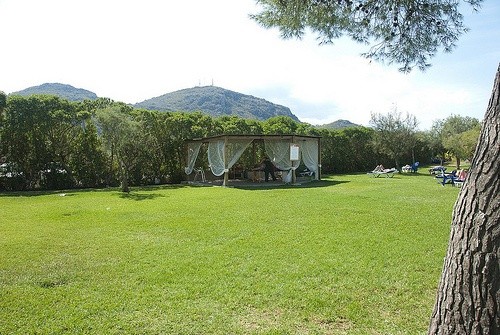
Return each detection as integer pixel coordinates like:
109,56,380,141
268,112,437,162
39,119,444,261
428,166,469,188
367,161,419,178
299,169,314,176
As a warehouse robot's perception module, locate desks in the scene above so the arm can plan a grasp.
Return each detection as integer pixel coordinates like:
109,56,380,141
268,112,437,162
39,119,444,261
252,166,284,181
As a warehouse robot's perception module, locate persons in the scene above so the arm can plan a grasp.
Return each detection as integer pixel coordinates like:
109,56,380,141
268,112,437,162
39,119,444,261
374,165,395,172
259,152,277,183
457,169,468,180
